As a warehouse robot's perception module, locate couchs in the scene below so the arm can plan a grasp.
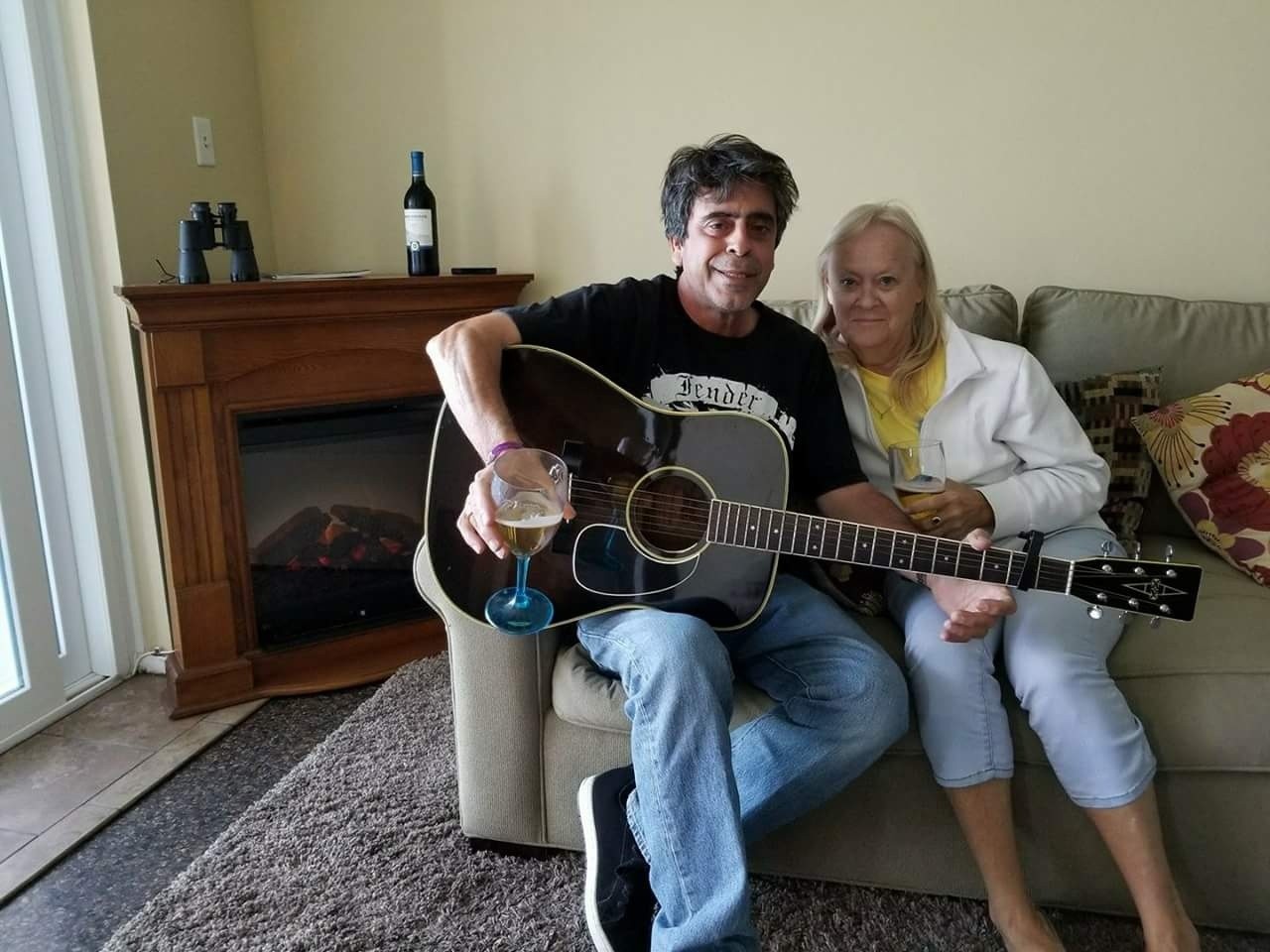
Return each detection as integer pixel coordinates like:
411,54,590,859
411,282,1270,936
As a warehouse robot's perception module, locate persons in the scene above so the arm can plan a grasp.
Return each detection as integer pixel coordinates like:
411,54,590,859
807,202,1202,952
425,134,1018,952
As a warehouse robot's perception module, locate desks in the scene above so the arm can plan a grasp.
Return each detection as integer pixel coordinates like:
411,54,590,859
109,273,535,721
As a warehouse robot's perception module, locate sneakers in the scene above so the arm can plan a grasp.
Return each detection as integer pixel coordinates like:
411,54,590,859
577,763,659,952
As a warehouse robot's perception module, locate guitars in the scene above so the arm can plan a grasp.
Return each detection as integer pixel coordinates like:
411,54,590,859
423,343,1203,635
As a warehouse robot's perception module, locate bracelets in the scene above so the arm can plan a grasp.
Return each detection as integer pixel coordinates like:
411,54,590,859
486,441,524,466
916,573,928,586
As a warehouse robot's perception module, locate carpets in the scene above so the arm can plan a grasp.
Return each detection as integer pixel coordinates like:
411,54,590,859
97,646,1270,952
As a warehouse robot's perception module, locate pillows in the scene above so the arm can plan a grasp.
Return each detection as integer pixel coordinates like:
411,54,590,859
1052,361,1162,565
1131,368,1270,590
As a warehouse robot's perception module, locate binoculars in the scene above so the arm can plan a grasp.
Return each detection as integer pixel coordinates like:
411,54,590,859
178,201,260,285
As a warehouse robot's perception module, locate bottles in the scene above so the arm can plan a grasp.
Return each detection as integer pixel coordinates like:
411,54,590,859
403,150,440,277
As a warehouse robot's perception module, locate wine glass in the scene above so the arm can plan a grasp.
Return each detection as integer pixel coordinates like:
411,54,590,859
487,447,568,638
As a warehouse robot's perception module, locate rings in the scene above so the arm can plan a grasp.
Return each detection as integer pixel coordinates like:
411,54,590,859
466,512,473,523
928,514,943,526
